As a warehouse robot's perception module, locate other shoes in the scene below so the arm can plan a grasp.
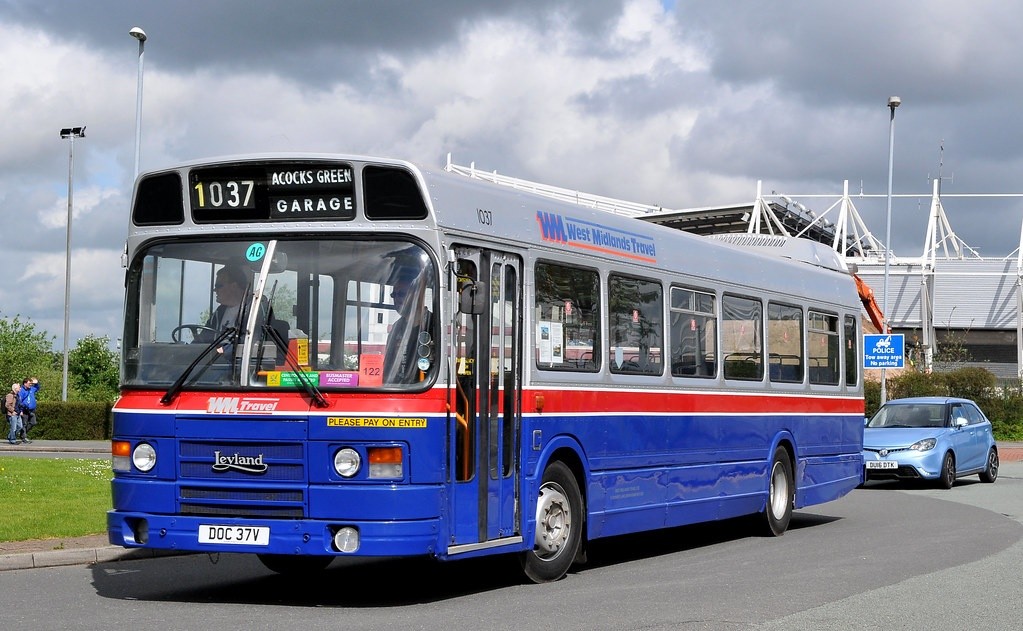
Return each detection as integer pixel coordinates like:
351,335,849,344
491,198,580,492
10,439,20,445
22,438,32,444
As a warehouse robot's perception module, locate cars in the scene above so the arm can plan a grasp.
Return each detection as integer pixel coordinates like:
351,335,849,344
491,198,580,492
861,394,1001,489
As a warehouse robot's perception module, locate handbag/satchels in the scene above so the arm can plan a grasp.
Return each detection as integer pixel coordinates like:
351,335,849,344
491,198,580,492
14,402,23,413
23,406,30,415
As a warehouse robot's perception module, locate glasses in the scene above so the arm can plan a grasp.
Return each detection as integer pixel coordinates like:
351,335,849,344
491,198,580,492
390,290,414,298
214,283,233,289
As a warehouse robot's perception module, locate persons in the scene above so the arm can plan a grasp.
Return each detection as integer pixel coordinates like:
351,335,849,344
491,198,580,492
191,266,275,344
382,274,433,383
5,383,32,444
18,377,41,444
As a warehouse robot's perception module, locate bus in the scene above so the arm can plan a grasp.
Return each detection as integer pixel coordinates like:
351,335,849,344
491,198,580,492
99,149,867,581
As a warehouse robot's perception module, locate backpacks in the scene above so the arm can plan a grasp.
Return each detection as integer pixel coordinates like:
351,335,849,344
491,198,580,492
1,393,14,414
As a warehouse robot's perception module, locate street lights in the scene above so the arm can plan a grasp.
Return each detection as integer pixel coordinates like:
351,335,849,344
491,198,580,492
879,94,900,421
59,125,88,402
127,25,147,186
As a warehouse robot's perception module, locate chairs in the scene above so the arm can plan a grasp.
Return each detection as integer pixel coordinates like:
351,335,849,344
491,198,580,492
579,350,835,383
